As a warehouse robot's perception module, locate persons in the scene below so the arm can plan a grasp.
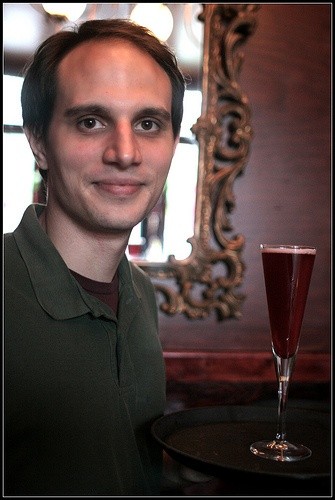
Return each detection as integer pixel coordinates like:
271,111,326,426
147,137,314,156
3,17,225,496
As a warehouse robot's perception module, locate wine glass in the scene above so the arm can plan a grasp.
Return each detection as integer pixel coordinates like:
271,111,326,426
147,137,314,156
248,243,316,461
